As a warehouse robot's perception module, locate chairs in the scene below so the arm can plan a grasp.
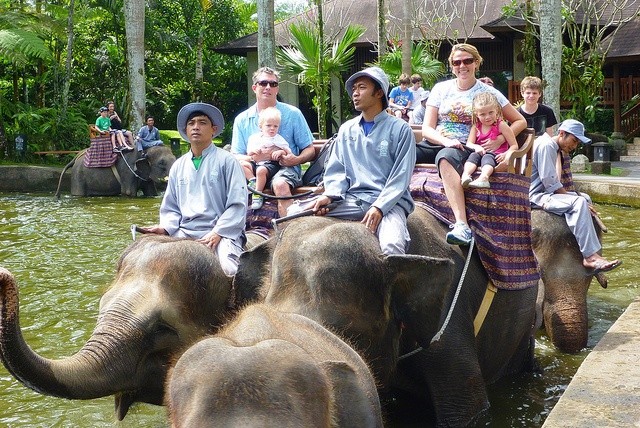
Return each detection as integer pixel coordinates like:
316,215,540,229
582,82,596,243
248,127,535,205
90,125,110,138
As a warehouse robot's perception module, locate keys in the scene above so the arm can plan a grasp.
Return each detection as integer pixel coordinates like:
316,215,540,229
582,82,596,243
355,200,364,212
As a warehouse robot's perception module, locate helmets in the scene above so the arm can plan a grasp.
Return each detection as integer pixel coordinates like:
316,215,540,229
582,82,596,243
99,107,109,113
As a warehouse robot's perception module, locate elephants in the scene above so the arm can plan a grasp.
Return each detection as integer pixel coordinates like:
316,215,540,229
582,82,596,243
54,146,177,198
164,298,384,427
234,201,545,427
530,208,608,354
0,225,282,420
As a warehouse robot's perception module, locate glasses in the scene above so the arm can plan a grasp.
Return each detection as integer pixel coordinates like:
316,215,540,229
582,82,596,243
108,102,114,104
452,58,476,66
255,81,278,87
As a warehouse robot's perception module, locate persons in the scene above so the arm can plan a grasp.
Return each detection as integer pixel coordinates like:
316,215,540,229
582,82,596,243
233,66,317,220
107,101,135,150
417,42,529,247
95,106,127,153
239,107,292,210
508,75,558,146
529,118,621,287
136,117,164,156
458,93,519,189
286,66,418,259
143,102,247,309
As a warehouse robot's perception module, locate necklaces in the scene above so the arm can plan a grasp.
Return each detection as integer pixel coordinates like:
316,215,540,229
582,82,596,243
455,80,477,91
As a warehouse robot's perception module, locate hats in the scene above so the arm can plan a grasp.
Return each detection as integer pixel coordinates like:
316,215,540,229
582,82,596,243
558,119,592,144
345,66,389,109
177,103,224,143
420,91,430,102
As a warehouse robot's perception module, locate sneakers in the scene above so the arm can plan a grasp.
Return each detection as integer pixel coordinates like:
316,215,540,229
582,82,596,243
113,147,122,152
118,145,127,151
124,145,134,151
247,181,256,194
251,193,263,210
461,177,473,188
446,222,471,245
469,181,491,189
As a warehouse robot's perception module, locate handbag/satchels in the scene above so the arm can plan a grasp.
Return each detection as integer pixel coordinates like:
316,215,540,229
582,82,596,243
110,118,121,130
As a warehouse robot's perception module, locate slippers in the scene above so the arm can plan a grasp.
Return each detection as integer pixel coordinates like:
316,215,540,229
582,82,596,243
586,260,622,277
